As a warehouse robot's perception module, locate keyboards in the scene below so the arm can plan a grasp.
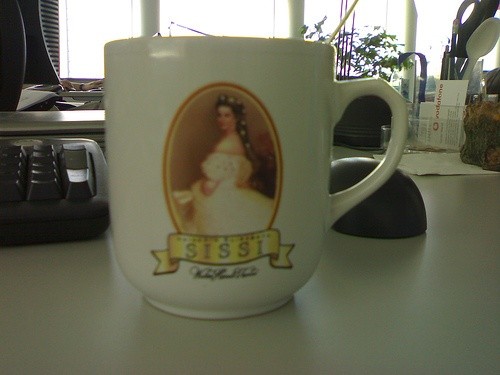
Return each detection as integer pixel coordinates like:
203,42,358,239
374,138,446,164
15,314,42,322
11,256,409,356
0,137,109,247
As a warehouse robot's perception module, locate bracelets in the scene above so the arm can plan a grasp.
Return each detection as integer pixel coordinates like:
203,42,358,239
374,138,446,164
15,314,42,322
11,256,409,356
80,84,87,92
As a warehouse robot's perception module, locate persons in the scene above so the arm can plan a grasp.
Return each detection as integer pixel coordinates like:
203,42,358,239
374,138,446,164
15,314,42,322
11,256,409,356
54,78,101,110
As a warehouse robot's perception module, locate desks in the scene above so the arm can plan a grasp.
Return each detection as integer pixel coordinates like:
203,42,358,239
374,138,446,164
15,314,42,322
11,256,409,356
0,118,499,374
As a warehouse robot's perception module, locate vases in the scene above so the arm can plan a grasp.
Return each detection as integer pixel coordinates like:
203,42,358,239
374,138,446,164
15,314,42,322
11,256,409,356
296,16,416,151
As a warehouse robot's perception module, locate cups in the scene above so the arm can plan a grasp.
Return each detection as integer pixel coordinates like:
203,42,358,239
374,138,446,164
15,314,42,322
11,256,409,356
104,36,408,320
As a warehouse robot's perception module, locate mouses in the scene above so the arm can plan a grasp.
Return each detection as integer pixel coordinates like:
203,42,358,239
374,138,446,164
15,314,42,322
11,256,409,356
328,157,427,240
